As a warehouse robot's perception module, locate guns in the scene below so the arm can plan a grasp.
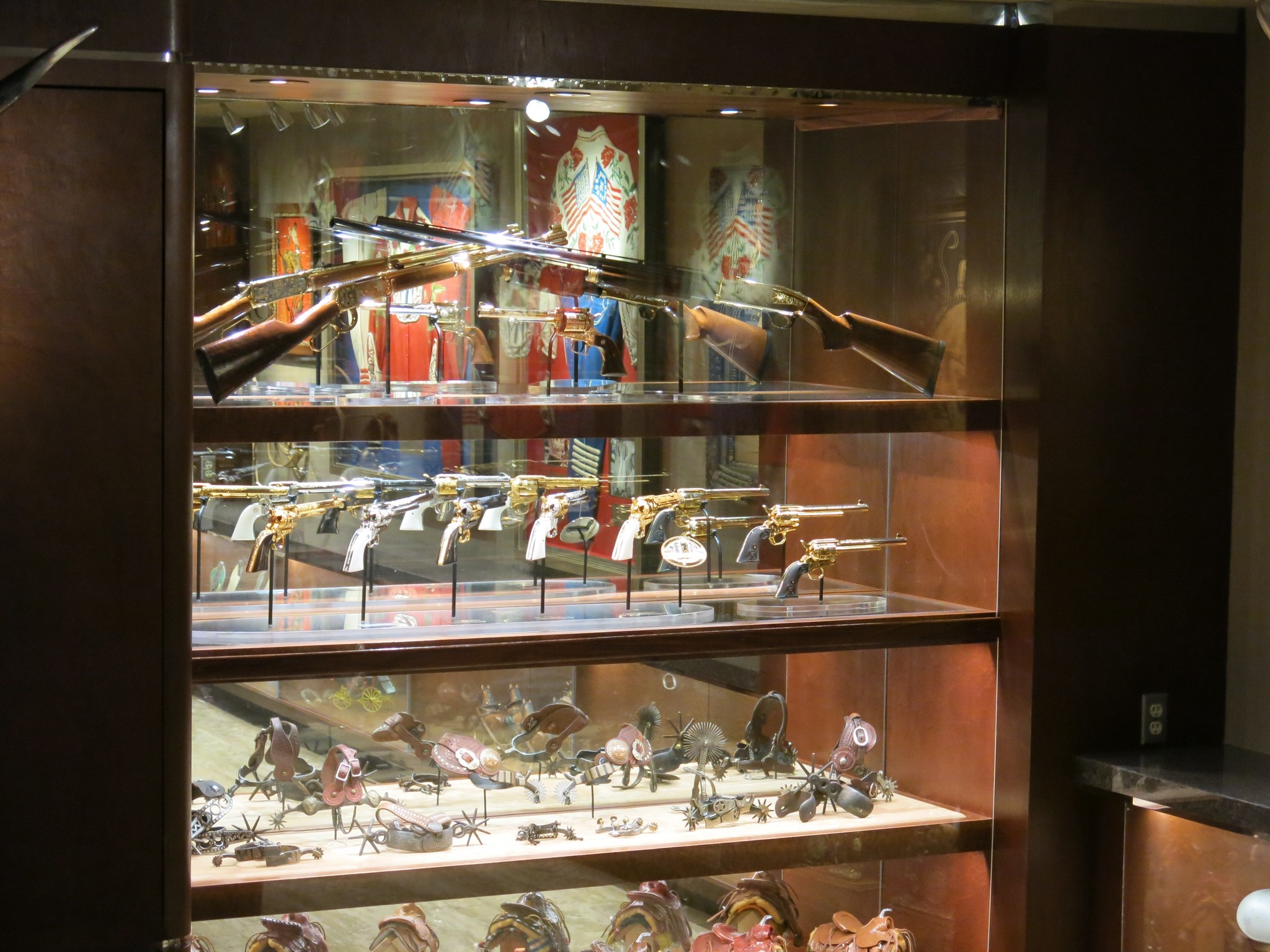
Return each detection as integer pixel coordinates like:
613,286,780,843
190,212,948,406
191,472,910,599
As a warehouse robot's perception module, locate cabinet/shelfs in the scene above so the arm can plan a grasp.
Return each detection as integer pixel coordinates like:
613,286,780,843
178,0,1080,952
